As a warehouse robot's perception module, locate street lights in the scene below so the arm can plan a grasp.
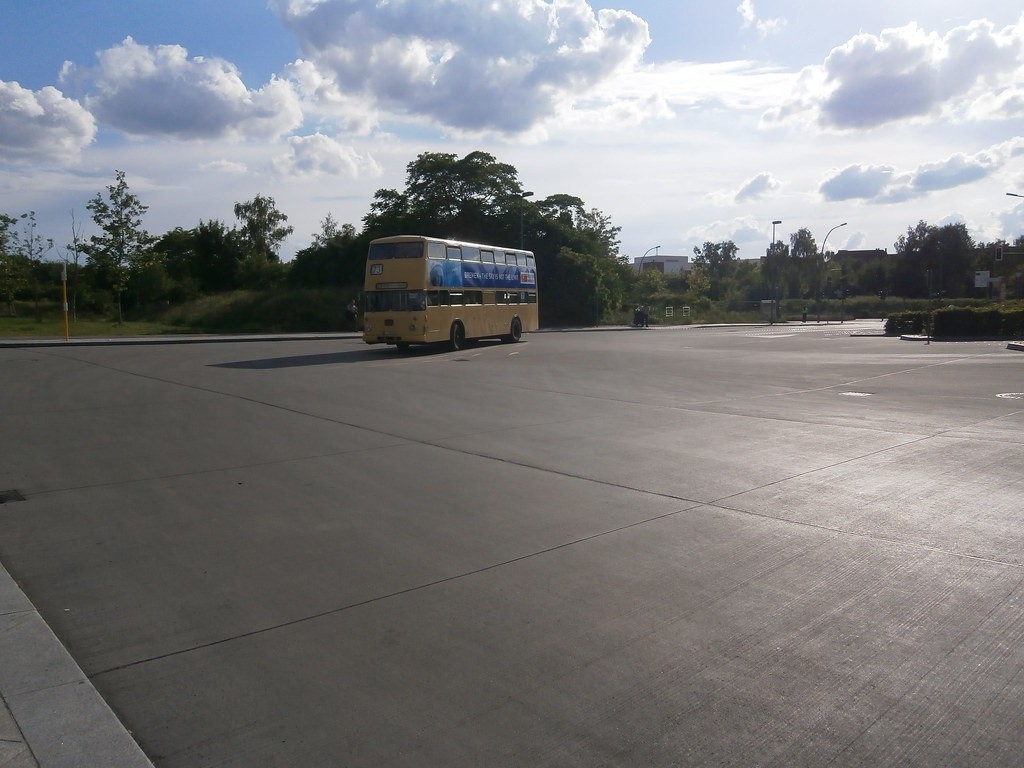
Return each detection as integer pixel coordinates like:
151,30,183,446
817,222,848,324
770,220,782,324
638,246,661,274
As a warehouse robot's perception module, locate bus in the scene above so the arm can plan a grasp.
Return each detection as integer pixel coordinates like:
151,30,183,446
361,235,539,352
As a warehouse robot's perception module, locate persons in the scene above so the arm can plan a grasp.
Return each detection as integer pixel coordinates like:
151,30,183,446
345,299,358,332
642,303,650,327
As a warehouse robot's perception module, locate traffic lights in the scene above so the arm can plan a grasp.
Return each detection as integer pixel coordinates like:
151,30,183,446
995,247,1003,261
